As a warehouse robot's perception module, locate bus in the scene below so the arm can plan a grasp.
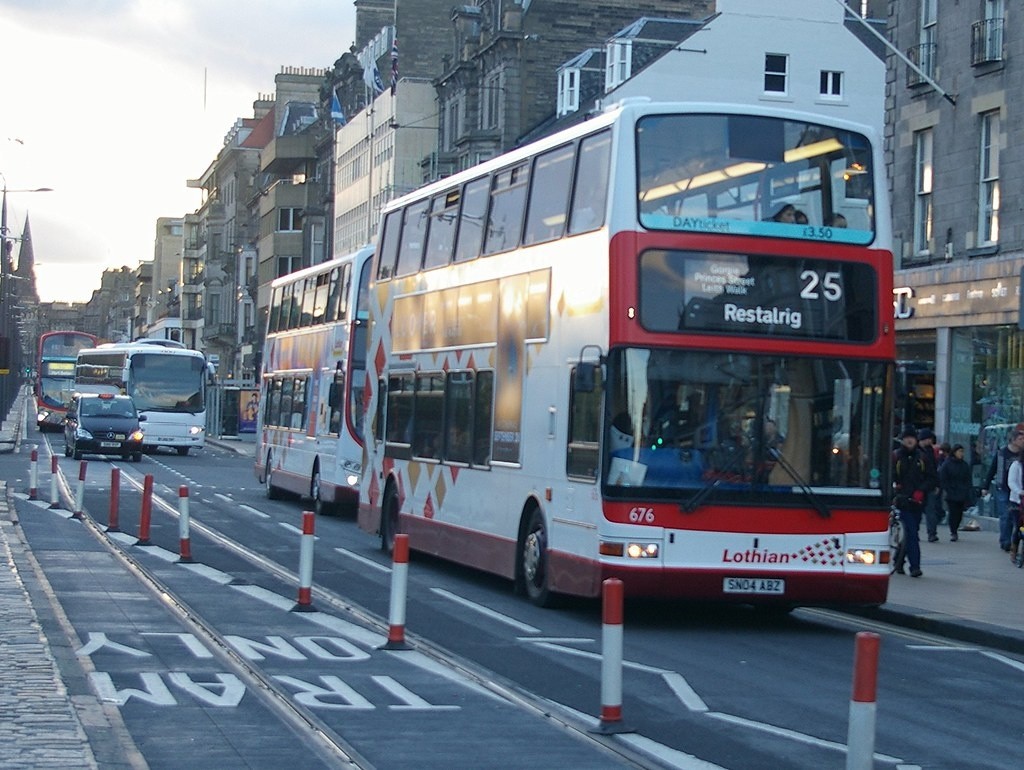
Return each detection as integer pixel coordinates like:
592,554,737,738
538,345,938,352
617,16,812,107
31,330,99,432
354,98,902,617
252,241,383,517
76,338,217,454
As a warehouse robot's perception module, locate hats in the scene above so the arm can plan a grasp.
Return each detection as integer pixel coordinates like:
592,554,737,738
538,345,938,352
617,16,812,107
952,444,963,454
901,425,917,439
917,428,933,439
939,442,950,450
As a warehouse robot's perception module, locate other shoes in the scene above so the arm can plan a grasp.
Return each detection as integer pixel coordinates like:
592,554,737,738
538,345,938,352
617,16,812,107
1000,543,1011,552
928,535,939,542
1009,551,1017,563
950,534,959,541
893,562,905,574
911,569,922,577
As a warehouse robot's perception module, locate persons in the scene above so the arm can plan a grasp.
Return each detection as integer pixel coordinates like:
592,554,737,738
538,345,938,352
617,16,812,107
911,427,940,543
762,200,848,229
930,432,942,462
981,430,1024,551
245,393,259,419
1007,445,1024,563
936,441,953,525
940,443,972,542
679,390,786,461
890,424,933,577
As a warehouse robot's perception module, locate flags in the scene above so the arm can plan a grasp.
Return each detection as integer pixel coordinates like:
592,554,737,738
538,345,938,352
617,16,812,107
367,42,386,93
330,86,346,125
390,24,399,97
362,45,372,89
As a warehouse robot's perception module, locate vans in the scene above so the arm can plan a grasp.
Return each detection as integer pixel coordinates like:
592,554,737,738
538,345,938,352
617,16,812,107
62,391,148,461
